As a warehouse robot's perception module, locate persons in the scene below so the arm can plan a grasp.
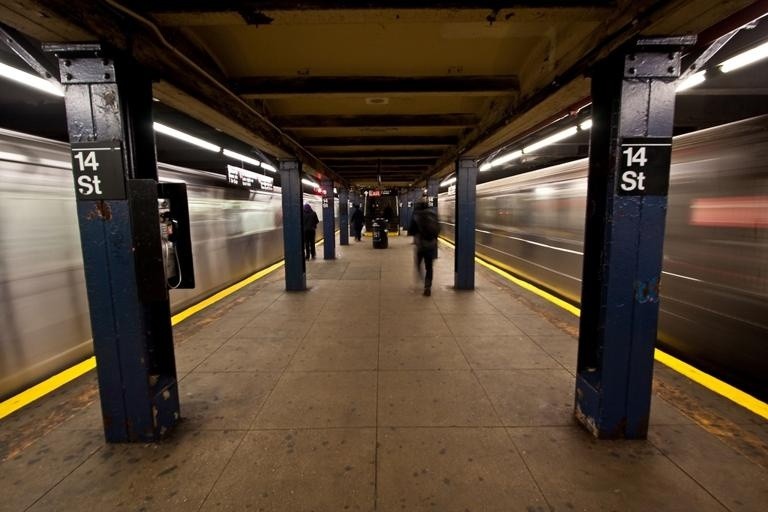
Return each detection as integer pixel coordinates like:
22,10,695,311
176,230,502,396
303,203,319,260
383,201,393,223
407,196,441,297
351,205,366,242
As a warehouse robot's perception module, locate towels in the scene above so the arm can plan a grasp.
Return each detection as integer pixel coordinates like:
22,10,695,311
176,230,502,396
372,221,388,249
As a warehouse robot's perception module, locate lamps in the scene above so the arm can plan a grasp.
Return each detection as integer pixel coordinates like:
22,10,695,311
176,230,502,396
261,162,279,174
676,69,706,93
579,118,592,131
223,149,260,167
523,125,577,154
0,62,65,97
717,42,768,74
490,150,522,168
302,178,320,188
153,121,221,153
440,177,457,187
478,163,491,172
